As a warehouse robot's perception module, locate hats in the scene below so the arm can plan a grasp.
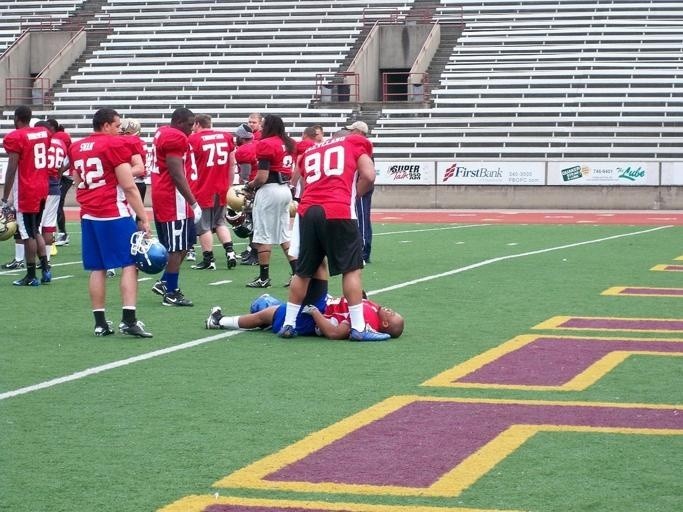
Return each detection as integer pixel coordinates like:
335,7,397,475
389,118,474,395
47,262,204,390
346,121,369,133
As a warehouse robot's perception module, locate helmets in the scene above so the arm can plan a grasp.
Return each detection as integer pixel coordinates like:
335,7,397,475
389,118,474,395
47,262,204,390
130,232,169,274
0,204,18,241
121,118,142,135
225,184,253,238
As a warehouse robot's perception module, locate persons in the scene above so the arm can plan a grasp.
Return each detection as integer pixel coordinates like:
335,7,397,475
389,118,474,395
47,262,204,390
67,109,405,340
2,105,74,285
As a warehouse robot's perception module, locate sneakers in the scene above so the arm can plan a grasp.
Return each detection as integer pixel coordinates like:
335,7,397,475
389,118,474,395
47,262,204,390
185,245,259,269
283,273,294,287
119,323,154,341
106,269,116,278
349,324,391,340
280,326,297,338
247,276,272,289
41,271,52,283
13,273,39,286
162,291,194,307
205,305,225,330
2,232,70,268
152,279,168,294
95,326,116,339
